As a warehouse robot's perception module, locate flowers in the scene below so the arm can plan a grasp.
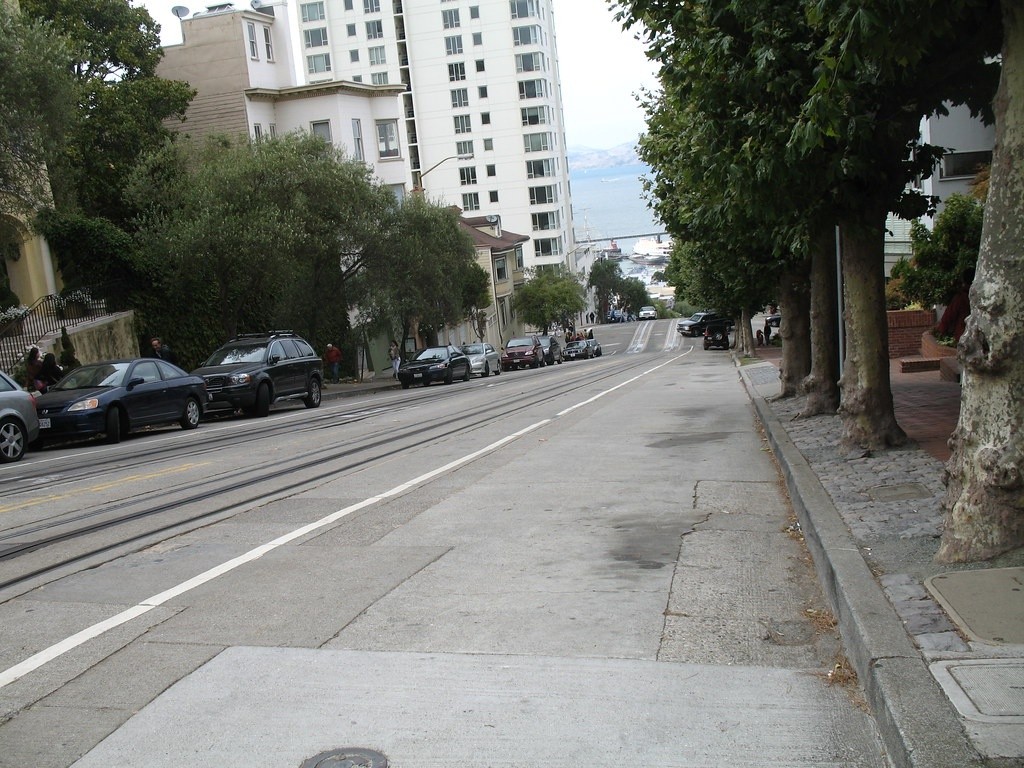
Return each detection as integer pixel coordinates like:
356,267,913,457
0,304,29,328
55,291,92,314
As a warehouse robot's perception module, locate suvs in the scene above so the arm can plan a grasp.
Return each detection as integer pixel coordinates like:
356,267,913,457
702,324,730,350
539,336,563,365
500,334,545,371
188,329,325,422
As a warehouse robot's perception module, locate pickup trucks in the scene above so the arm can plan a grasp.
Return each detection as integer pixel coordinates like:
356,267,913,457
677,312,736,337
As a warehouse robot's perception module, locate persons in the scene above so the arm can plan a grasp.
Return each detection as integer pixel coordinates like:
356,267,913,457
620,306,625,323
575,329,593,341
756,330,764,346
627,304,635,321
25,347,41,391
565,332,574,343
590,312,594,323
326,344,343,384
586,313,589,323
35,353,64,385
610,306,616,320
388,340,401,380
764,323,771,345
149,337,171,362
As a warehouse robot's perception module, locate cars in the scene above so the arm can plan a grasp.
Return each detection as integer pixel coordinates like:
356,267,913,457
638,306,658,321
33,358,208,448
766,315,782,328
0,367,40,464
458,343,501,378
398,344,472,389
562,339,602,360
608,310,622,322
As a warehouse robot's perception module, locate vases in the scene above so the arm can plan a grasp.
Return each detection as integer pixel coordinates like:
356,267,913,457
62,305,84,318
6,322,22,337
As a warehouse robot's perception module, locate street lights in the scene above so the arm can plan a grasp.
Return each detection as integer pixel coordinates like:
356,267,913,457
417,152,474,203
